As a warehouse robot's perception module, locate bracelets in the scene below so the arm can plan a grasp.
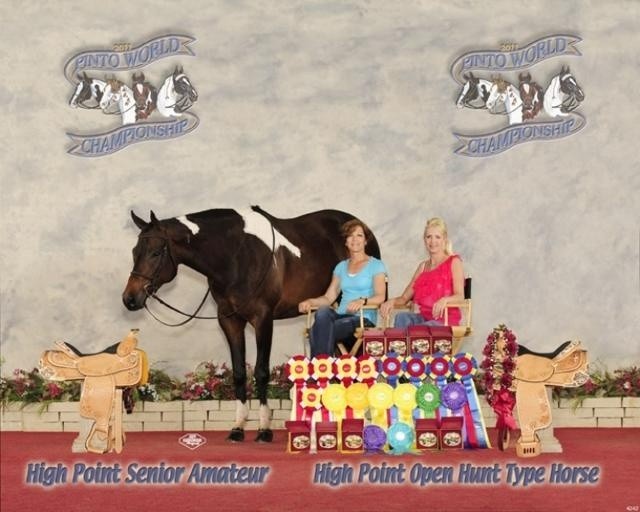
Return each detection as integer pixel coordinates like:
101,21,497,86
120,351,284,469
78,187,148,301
360,296,369,306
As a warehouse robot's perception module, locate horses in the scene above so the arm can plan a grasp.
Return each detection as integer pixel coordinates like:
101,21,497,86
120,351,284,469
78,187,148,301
122,204,382,444
455,65,584,125
68,64,198,126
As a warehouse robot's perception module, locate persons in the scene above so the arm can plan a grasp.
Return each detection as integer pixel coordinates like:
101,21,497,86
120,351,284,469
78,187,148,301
378,216,468,332
298,220,388,360
